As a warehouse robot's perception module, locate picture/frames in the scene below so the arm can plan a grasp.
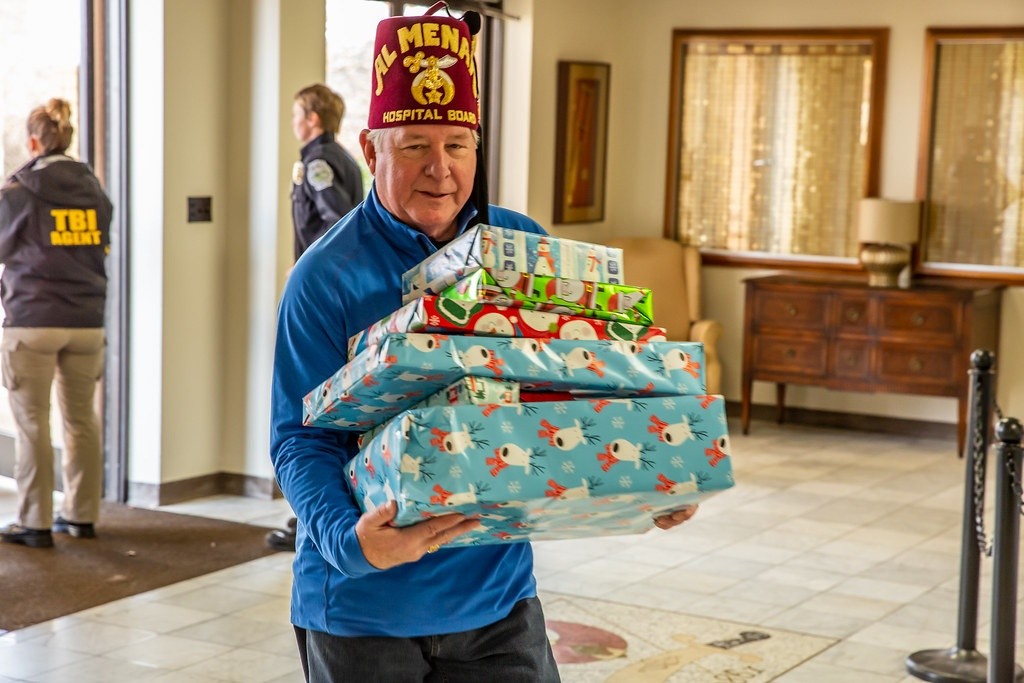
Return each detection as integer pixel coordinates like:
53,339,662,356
554,60,611,223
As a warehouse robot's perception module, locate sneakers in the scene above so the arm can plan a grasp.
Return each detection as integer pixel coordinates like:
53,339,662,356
0,519,94,549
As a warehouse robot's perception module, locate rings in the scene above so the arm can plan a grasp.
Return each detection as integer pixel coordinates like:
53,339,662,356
428,544,440,553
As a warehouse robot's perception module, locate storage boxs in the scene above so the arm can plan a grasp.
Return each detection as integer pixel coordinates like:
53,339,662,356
302,224,735,548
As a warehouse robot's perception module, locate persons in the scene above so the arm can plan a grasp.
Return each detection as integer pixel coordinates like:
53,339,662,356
271,99,696,683
1,100,114,547
265,85,361,550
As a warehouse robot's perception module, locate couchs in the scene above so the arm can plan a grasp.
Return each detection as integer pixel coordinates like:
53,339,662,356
600,234,721,395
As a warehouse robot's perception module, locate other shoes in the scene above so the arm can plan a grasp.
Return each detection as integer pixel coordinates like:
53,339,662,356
264,518,296,551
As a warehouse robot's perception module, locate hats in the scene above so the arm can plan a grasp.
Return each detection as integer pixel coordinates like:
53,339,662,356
367,15,480,131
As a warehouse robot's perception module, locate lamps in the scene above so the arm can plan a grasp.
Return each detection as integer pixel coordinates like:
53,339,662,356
860,198,921,287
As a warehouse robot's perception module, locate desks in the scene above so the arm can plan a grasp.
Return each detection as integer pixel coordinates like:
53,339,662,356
742,276,1009,459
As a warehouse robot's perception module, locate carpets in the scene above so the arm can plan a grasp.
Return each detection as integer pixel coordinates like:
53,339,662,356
1,498,287,631
